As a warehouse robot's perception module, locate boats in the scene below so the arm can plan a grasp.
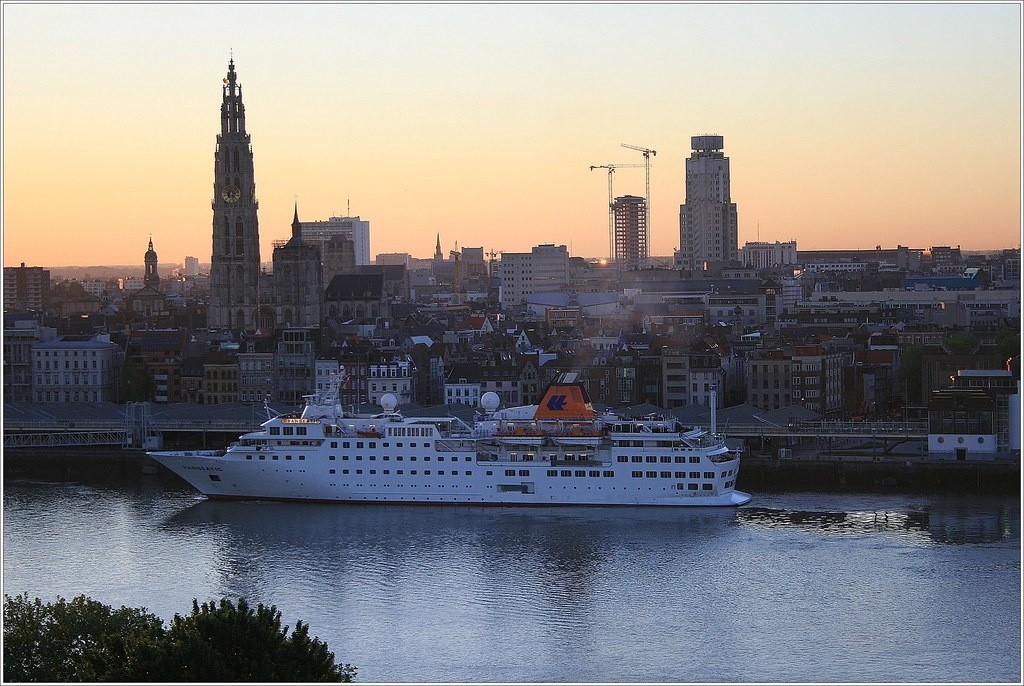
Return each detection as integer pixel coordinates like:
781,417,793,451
145,367,753,507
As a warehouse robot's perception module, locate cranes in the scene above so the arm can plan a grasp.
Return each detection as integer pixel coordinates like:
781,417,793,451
590,163,653,270
620,142,657,257
